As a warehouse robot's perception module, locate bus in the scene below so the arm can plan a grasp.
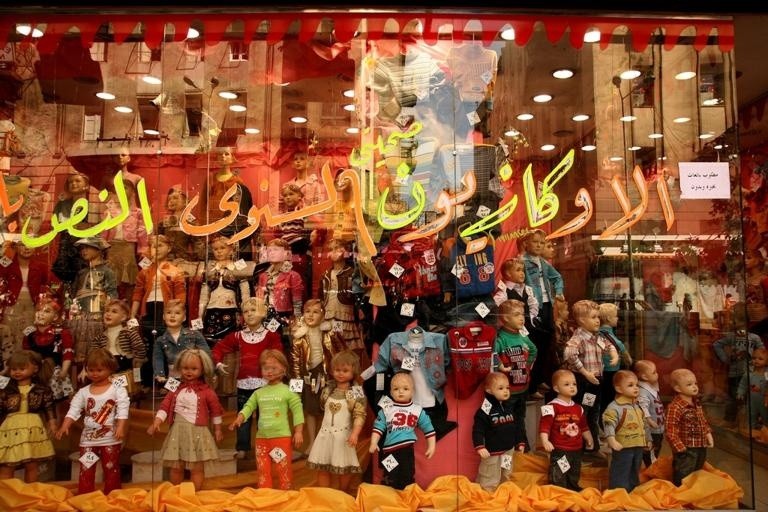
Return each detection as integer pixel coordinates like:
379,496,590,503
587,250,738,318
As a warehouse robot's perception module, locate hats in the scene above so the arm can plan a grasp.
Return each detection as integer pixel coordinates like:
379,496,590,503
74,235,111,250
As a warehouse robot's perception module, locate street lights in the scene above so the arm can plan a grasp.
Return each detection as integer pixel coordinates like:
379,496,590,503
305,116,330,178
182,74,220,284
611,73,655,372
723,123,755,511
450,71,469,328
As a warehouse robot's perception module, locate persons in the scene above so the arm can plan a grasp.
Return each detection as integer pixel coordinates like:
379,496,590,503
599,370,655,493
0,352,57,481
538,370,594,492
56,349,131,494
368,374,436,492
229,349,305,491
1,144,768,468
147,351,225,490
469,371,527,488
666,368,714,486
308,349,367,492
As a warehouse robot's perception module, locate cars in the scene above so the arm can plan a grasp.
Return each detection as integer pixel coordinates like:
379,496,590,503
446,301,496,325
